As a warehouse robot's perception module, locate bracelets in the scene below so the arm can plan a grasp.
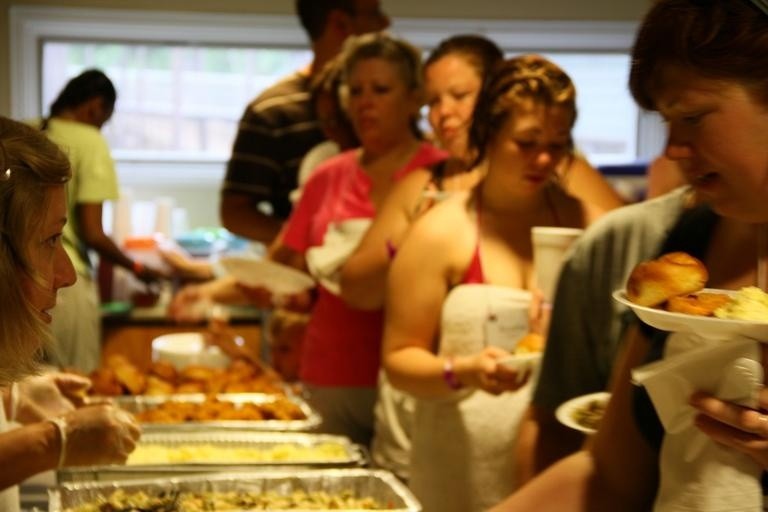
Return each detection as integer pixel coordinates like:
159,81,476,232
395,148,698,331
444,361,465,395
128,262,143,276
10,381,22,416
45,413,71,472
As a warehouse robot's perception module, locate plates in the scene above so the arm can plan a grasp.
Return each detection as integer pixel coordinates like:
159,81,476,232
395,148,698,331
556,391,613,434
496,350,547,378
612,288,768,341
218,253,313,295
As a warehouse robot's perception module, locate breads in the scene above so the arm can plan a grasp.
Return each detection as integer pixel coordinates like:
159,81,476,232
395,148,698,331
626,250,708,309
669,291,733,318
132,394,305,422
86,354,283,394
515,333,542,355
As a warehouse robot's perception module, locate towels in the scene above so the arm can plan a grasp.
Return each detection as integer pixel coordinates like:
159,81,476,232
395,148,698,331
307,223,363,277
629,325,765,512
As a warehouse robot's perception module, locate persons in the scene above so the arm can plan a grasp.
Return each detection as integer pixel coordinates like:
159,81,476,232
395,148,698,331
1,116,78,382
488,2,766,511
370,57,606,510
234,34,451,440
339,36,627,315
169,44,360,383
511,188,702,488
1,366,140,489
219,0,391,246
40,69,167,374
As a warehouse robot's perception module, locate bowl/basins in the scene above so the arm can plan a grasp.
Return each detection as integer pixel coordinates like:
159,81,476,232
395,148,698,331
152,334,243,371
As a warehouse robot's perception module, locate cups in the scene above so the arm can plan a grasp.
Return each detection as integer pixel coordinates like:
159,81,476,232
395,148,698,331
530,226,580,298
